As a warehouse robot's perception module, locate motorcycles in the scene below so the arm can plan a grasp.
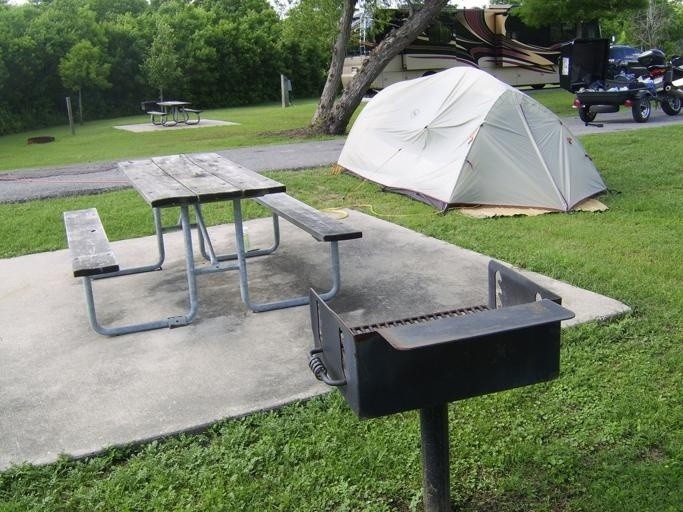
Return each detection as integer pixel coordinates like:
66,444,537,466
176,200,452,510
576,43,683,123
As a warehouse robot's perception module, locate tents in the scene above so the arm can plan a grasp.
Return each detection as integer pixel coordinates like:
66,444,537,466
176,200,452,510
337,66,608,214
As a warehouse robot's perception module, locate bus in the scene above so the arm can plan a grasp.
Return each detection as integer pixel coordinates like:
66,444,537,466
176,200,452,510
340,2,601,96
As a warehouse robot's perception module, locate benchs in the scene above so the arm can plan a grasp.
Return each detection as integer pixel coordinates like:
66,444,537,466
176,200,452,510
145,108,203,128
65,207,122,336
257,192,363,296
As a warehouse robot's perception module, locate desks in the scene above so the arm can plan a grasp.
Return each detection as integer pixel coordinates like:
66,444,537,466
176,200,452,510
156,101,192,125
117,151,288,325
